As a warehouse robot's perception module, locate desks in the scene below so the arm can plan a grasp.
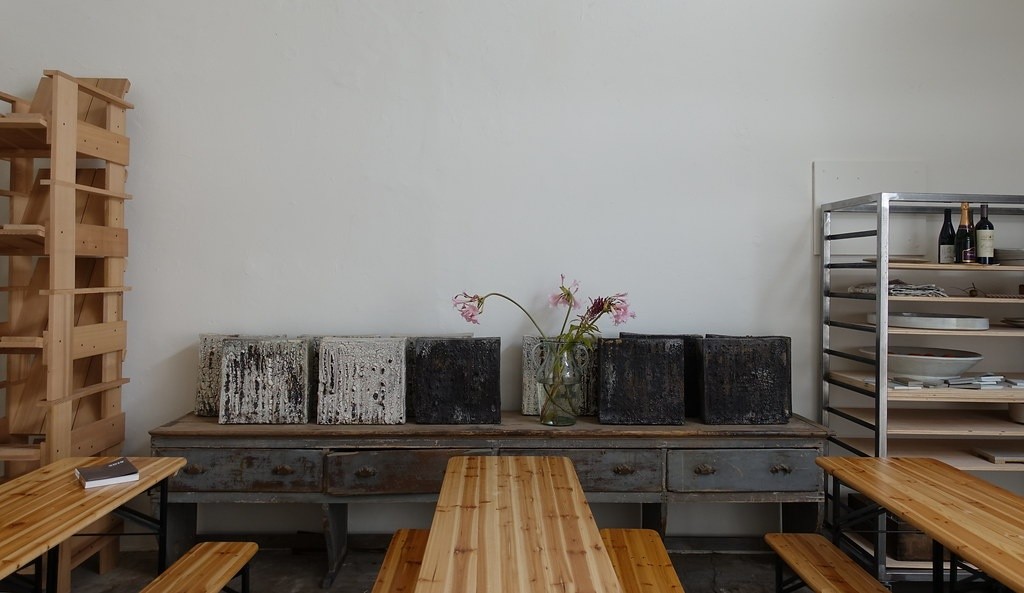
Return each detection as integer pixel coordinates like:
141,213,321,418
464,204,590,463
411,455,625,593
815,457,1024,593
0,455,188,593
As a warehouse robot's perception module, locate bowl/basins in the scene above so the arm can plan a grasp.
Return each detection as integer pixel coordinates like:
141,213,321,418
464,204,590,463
860,345,983,376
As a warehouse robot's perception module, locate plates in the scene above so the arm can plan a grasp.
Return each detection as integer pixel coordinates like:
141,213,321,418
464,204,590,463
863,259,931,264
1000,318,1024,326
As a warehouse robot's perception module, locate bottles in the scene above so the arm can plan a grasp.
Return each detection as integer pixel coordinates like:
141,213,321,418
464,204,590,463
974,205,994,265
956,202,975,263
938,210,955,263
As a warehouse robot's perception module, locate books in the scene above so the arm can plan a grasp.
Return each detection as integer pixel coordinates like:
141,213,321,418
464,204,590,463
75,456,142,489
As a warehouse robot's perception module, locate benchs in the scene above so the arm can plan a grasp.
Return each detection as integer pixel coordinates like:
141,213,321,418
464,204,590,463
600,528,684,593
765,533,893,593
140,542,260,593
370,529,430,593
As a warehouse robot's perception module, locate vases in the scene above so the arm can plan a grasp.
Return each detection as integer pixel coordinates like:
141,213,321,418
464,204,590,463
532,341,590,426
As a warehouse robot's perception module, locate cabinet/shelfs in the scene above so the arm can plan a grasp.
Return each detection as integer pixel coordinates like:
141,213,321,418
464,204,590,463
0,70,130,593
819,192,1024,593
144,407,836,578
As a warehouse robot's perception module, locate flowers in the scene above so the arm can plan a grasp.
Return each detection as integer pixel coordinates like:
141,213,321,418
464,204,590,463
450,273,636,425
